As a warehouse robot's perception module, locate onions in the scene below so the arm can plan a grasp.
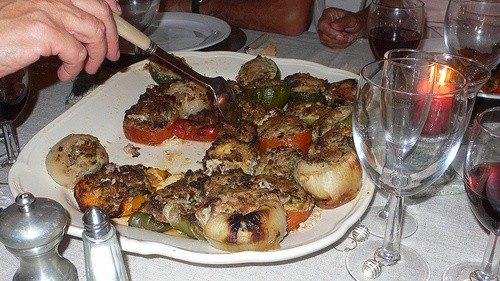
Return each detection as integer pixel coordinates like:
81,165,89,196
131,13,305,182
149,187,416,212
45,133,110,188
203,196,287,251
293,146,362,208
167,79,216,118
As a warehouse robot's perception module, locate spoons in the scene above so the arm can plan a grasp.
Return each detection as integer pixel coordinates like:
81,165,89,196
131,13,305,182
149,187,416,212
113,13,241,127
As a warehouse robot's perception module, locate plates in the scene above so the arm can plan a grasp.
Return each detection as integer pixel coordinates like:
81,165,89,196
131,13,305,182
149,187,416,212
144,13,231,52
420,37,500,100
7,48,375,265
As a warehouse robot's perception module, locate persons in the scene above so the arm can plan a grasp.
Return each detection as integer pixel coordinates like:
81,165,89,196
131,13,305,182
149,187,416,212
0,0,124,82
317,0,500,49
158,0,314,35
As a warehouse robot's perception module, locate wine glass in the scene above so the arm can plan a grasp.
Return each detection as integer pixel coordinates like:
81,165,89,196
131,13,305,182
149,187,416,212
115,0,161,62
442,106,500,281
444,0,500,143
358,48,437,241
345,58,468,281
399,52,491,186
0,68,31,184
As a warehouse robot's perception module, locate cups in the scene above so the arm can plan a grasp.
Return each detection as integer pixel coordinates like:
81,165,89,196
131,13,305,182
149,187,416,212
367,0,424,60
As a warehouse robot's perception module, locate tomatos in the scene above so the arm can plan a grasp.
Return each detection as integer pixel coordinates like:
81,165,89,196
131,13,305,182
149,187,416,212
123,111,219,145
258,130,313,156
287,207,313,231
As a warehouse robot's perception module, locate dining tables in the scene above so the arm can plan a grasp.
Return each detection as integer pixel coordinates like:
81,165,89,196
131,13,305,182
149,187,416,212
0,31,500,281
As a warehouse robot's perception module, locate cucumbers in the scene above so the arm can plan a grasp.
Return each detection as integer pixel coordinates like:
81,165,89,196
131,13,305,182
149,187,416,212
237,56,371,133
149,62,179,85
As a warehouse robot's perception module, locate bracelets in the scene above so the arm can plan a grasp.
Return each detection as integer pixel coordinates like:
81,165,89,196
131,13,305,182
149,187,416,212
192,0,200,14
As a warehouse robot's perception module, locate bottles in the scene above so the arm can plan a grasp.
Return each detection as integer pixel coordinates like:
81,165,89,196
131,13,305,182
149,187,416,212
82,206,129,281
0,192,79,281
